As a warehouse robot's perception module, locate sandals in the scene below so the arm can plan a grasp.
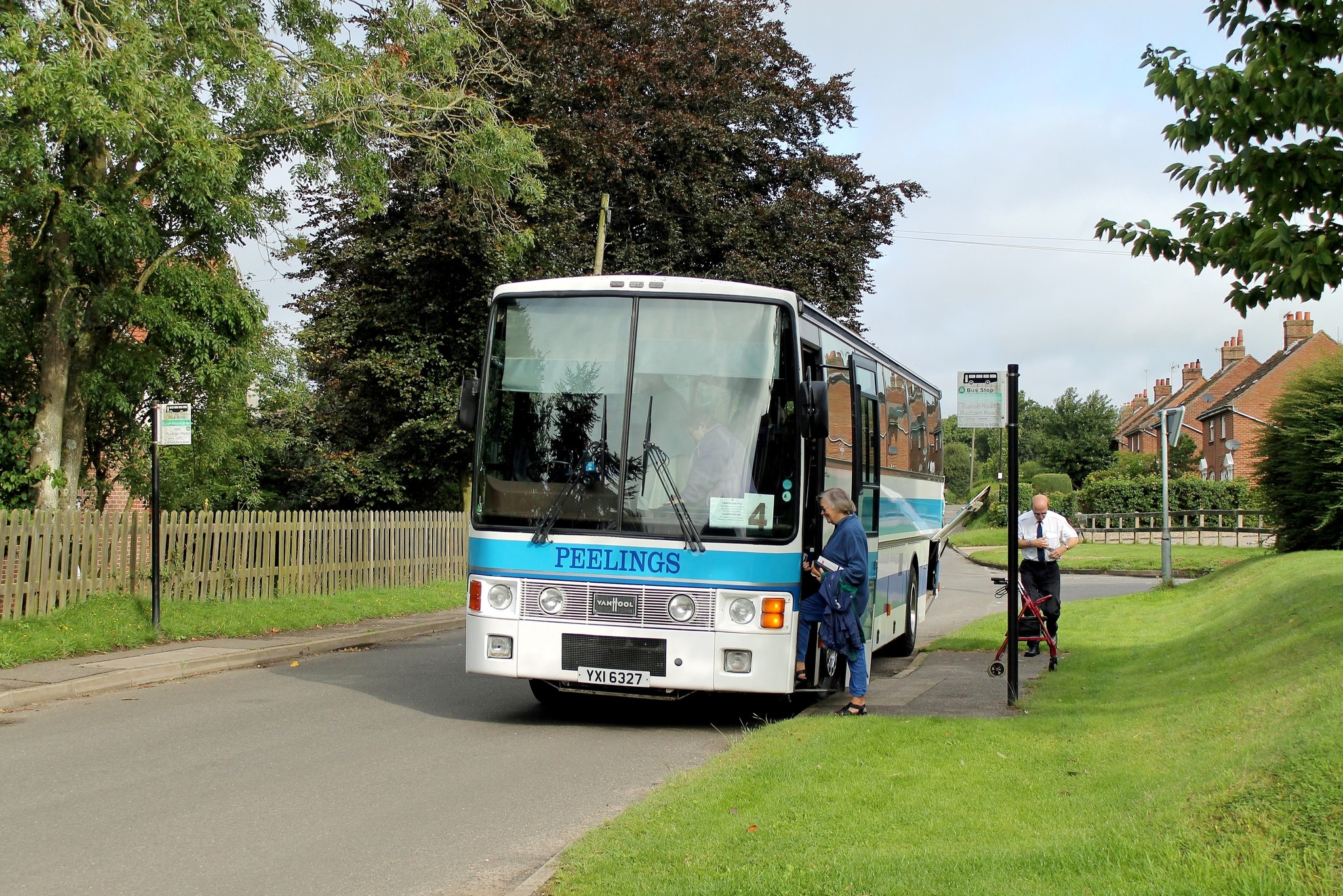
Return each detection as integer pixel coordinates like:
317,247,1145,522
796,670,807,682
834,702,867,716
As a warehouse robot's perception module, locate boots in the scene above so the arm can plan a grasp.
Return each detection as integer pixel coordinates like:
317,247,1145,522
1024,646,1040,657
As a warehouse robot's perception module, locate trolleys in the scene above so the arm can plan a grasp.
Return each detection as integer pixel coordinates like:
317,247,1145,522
987,577,1057,679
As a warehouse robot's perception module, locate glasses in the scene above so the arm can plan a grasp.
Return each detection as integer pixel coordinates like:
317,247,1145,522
820,506,831,511
1034,512,1046,514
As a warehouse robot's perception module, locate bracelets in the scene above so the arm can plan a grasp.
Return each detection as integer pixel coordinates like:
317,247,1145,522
1028,540,1032,547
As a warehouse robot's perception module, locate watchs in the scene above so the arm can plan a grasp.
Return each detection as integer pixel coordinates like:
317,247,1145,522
1064,544,1068,550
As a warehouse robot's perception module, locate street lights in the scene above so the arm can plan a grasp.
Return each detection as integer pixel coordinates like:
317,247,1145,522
969,452,971,478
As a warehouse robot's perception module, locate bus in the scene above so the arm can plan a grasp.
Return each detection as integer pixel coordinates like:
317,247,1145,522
464,275,991,726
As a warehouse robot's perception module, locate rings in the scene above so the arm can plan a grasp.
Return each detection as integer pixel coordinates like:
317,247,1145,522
1055,556,1057,558
672,500,673,503
804,569,806,571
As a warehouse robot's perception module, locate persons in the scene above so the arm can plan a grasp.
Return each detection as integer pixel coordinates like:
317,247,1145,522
661,406,757,507
634,374,695,516
727,384,762,447
793,488,870,716
1018,494,1078,657
